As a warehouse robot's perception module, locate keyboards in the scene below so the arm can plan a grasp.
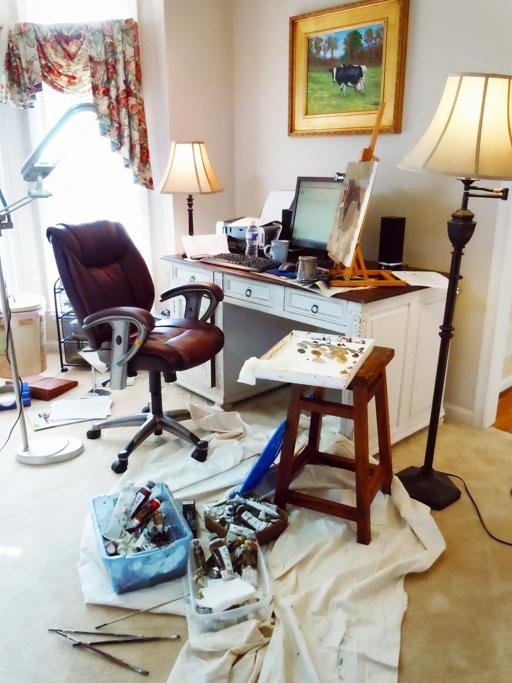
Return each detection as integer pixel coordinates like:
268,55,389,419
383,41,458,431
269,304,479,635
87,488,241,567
201,253,273,272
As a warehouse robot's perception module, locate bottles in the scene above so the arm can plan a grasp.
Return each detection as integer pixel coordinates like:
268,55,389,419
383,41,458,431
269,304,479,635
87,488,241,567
244,219,260,257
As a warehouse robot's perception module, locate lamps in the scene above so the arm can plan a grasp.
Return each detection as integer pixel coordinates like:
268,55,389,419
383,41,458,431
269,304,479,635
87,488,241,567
0,102,102,467
395,72,512,512
161,141,225,260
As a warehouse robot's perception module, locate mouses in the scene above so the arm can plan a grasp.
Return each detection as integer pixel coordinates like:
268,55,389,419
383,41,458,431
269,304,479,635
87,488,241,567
278,262,297,273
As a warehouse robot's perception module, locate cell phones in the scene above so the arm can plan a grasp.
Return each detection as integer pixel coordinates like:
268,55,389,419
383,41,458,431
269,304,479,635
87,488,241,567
190,254,209,260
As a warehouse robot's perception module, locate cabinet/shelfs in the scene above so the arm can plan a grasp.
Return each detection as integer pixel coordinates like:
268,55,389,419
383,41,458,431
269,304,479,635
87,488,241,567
53,276,94,371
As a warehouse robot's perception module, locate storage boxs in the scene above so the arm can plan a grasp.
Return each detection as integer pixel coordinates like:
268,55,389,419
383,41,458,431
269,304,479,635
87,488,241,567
188,537,273,634
93,484,194,595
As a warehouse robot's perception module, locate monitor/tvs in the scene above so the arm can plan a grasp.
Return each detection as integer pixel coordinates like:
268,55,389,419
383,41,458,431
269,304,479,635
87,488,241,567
290,176,344,269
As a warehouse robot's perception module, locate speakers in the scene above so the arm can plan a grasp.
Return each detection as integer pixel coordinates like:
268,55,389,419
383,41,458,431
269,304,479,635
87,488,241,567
281,209,292,240
377,216,406,266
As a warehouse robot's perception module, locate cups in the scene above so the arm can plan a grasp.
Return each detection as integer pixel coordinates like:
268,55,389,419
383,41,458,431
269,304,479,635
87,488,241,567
264,239,290,264
296,255,320,284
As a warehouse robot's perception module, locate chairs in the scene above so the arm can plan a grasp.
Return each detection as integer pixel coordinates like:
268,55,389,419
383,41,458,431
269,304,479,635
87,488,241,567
46,220,226,474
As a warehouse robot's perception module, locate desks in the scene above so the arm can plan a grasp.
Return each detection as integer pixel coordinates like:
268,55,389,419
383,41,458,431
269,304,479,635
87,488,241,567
160,252,462,457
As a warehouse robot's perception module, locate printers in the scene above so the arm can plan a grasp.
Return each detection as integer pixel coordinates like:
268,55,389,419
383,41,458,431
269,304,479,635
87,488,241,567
216,216,282,259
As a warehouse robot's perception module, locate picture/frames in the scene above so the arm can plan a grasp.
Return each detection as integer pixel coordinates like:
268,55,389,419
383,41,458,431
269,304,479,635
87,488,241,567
288,0,410,138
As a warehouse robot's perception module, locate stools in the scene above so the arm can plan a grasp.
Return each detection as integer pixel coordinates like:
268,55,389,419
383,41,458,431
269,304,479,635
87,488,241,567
274,347,396,544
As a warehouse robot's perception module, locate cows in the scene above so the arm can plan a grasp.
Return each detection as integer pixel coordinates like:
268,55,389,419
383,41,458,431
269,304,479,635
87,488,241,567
329,63,367,96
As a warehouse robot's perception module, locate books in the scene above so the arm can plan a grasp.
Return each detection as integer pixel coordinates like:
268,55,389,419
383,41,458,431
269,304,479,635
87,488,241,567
49,395,114,419
28,400,112,431
